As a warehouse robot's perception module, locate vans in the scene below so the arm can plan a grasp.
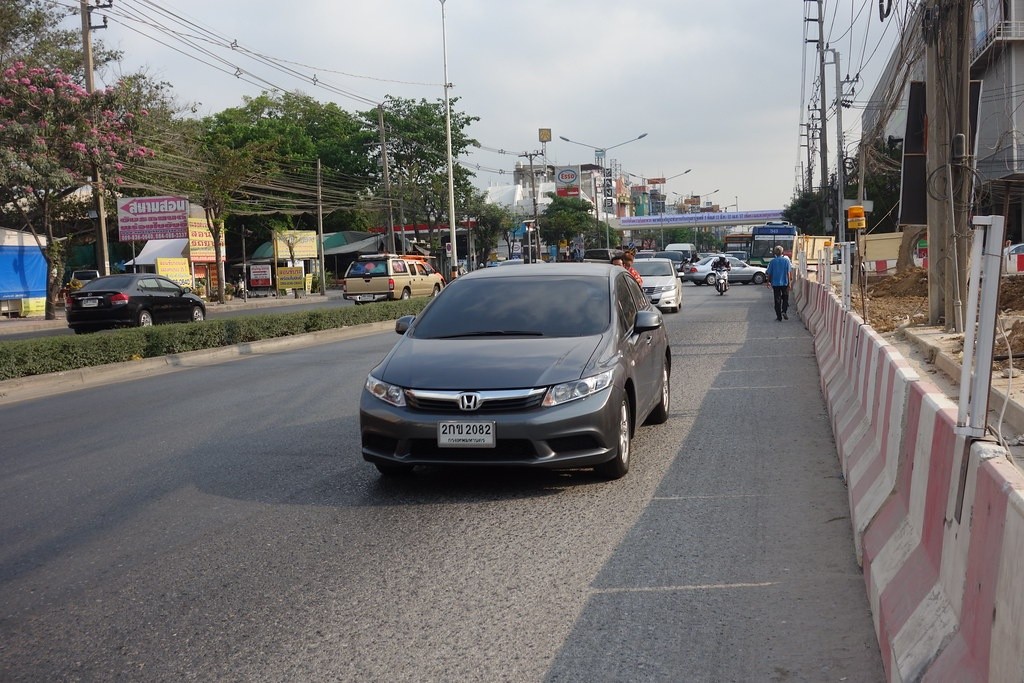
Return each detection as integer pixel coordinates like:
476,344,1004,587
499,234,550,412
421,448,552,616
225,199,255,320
665,243,697,263
71,269,99,290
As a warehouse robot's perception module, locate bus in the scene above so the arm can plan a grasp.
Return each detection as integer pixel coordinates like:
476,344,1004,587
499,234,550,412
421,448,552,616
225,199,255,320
725,232,752,263
748,220,802,268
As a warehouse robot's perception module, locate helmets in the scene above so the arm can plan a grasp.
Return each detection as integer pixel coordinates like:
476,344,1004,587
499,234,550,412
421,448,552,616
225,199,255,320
719,254,725,258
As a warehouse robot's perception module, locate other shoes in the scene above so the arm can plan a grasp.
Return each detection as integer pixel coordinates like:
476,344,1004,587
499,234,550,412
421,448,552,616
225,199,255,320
782,311,788,320
777,317,782,321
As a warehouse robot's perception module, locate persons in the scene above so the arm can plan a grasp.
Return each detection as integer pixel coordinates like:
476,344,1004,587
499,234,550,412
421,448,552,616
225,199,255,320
681,256,690,265
766,246,791,321
622,251,643,283
236,279,244,295
690,254,699,263
574,244,581,254
458,263,466,274
711,254,731,271
610,257,624,268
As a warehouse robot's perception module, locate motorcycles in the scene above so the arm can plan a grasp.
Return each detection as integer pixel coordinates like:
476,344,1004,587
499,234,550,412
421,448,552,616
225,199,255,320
232,274,245,299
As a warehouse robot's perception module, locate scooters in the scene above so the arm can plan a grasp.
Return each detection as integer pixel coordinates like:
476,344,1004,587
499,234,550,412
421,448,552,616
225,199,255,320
712,265,729,295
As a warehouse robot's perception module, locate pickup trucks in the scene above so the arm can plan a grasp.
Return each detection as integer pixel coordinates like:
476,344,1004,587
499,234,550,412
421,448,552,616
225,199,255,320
341,253,445,304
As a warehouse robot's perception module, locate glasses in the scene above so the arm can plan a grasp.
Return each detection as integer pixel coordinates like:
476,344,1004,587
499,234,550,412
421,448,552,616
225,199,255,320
623,262,631,267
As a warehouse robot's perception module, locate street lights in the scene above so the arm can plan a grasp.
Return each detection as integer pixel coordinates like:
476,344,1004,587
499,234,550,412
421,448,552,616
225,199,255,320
672,189,720,246
560,134,648,249
522,220,535,263
629,169,691,251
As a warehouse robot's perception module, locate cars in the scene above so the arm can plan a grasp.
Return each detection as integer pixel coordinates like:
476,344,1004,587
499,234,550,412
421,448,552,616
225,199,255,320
655,251,686,282
634,252,656,258
683,255,769,286
64,274,207,334
498,259,546,266
583,249,625,264
981,243,1023,281
358,260,673,480
631,258,685,313
726,251,748,265
